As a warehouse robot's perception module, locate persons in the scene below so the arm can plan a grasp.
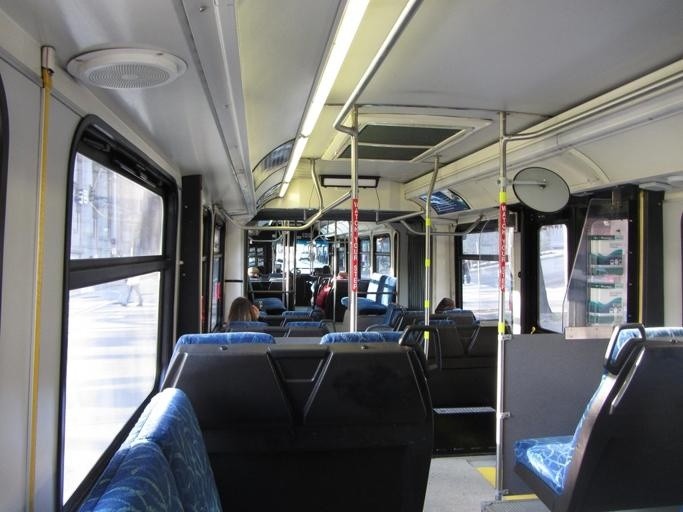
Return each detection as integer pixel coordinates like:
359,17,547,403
461,259,473,285
225,296,265,322
323,272,348,294
248,267,264,298
120,276,143,308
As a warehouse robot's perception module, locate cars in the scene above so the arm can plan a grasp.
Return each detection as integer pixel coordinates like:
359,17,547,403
298,250,310,261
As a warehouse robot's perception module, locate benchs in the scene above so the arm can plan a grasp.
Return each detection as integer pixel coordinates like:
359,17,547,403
74,323,442,511
171,271,510,409
512,324,682,511
